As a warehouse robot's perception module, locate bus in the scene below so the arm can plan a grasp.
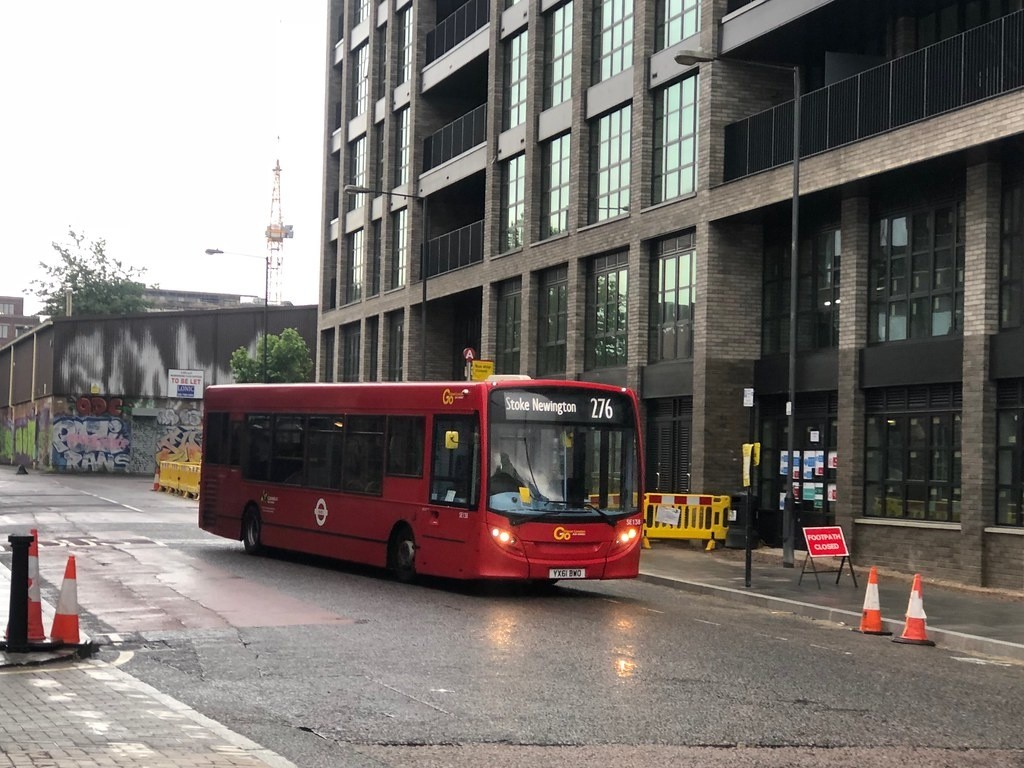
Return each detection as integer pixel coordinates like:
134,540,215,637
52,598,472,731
198,373,647,586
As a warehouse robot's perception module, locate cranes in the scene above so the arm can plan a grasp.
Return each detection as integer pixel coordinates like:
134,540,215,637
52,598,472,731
263,158,296,306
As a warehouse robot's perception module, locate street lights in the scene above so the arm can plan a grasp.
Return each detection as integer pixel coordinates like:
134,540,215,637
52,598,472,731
206,247,269,383
343,186,428,383
671,48,803,569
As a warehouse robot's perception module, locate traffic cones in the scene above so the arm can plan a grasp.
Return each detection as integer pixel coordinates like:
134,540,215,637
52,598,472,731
851,567,894,637
49,554,89,649
149,467,160,491
0,529,64,650
892,574,937,647
